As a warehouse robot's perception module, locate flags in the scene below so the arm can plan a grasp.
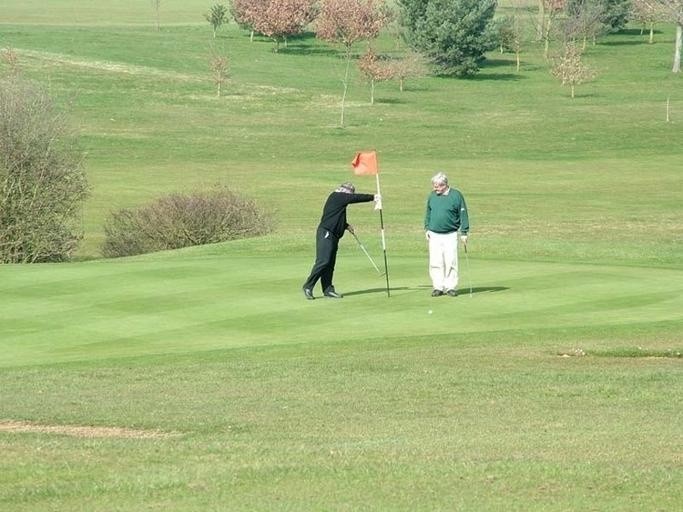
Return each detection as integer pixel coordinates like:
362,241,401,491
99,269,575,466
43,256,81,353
350,151,380,177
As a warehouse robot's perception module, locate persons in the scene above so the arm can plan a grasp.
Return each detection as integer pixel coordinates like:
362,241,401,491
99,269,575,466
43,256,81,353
300,180,382,300
422,172,469,297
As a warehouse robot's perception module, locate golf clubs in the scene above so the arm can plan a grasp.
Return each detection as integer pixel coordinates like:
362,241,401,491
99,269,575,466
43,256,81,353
463,244,473,298
352,231,386,277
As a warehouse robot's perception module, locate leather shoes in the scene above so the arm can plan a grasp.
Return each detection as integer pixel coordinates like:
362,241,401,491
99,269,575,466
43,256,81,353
431,289,443,297
446,289,457,297
303,285,315,299
324,288,343,298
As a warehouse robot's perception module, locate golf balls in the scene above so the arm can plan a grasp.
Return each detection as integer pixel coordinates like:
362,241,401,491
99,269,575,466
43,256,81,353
428,309,435,316
